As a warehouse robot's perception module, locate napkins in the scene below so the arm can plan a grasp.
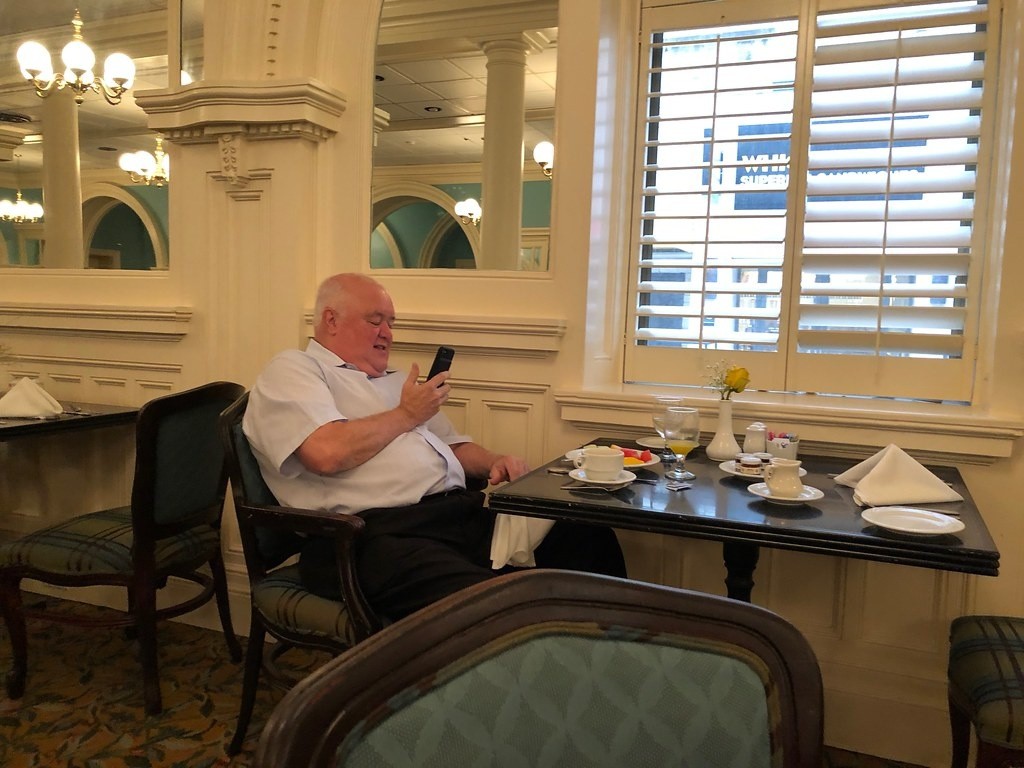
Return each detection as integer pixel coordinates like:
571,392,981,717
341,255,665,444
0,376,63,420
834,443,966,507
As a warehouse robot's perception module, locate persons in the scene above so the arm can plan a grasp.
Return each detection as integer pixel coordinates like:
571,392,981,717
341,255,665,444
240,274,626,636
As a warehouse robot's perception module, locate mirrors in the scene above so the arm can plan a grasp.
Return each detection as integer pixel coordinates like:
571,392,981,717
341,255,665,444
180,0,203,86
369,1,560,273
0,1,170,272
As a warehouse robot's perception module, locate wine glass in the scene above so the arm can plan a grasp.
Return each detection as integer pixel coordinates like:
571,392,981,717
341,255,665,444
664,407,700,481
652,396,683,460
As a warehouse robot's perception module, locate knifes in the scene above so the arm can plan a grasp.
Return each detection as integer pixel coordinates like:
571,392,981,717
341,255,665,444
548,467,659,483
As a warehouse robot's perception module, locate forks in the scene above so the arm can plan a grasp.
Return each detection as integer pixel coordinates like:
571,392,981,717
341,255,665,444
560,481,633,492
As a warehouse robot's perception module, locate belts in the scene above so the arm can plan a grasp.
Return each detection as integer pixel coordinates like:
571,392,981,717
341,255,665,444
422,489,461,502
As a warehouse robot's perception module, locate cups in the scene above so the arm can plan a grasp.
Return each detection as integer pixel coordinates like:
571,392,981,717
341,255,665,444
763,459,802,497
574,448,624,481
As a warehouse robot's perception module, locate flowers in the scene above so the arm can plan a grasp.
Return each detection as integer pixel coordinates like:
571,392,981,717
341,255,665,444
702,358,750,401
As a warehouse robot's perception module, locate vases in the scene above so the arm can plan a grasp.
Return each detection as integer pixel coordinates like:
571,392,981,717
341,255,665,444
705,399,742,462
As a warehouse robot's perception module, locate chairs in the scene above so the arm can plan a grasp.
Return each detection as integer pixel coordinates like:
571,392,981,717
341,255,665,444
949,616,1024,768
256,568,824,768
218,390,489,757
0,382,245,717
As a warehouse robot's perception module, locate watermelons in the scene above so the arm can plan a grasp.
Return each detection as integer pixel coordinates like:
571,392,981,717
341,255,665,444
621,447,652,462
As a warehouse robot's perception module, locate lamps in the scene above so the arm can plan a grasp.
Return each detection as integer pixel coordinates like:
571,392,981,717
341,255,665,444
16,8,136,106
454,197,483,226
0,188,44,224
532,141,554,181
118,137,169,189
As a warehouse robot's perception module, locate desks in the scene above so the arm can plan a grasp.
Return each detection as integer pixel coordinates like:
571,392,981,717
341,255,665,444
488,438,1000,603
0,400,140,443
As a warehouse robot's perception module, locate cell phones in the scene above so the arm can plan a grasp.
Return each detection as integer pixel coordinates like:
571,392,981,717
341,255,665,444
425,346,455,388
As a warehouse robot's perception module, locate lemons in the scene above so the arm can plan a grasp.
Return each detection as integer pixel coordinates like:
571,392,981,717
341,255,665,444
623,456,645,465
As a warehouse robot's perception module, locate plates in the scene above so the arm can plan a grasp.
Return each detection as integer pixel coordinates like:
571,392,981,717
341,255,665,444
568,467,637,488
861,506,965,538
636,437,701,450
747,482,825,505
719,460,808,482
565,448,661,467
